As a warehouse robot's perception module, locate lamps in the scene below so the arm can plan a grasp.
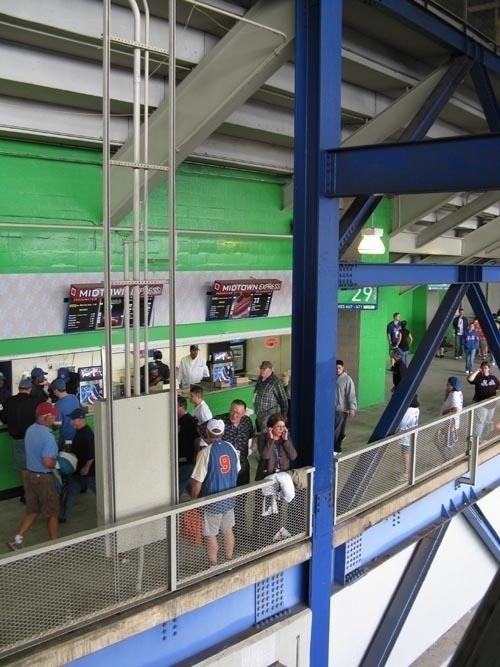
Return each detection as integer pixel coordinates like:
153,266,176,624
358,211,386,255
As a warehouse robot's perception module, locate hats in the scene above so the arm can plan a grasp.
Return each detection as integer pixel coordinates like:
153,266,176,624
190,345,200,352
449,377,462,391
392,347,404,357
66,408,85,418
149,363,160,370
35,402,61,416
0,368,70,392
259,361,273,369
207,419,225,436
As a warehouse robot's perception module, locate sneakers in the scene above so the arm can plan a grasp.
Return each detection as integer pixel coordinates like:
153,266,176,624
396,474,408,482
6,534,22,551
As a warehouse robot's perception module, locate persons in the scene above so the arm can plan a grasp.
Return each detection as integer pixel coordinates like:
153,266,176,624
0,365,97,553
139,342,301,568
467,361,500,440
432,377,463,465
392,394,420,482
392,321,413,369
333,360,357,457
386,313,403,371
391,348,407,392
452,308,500,375
